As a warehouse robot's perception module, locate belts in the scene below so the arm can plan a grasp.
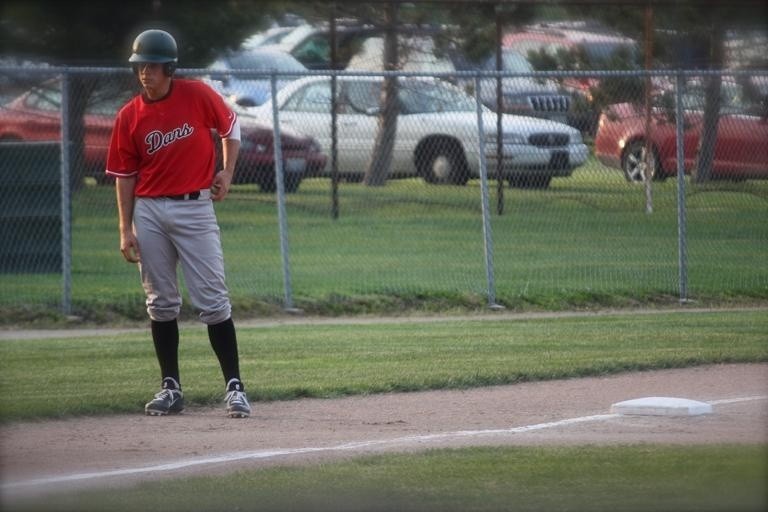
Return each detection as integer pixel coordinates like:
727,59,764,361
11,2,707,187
169,192,200,200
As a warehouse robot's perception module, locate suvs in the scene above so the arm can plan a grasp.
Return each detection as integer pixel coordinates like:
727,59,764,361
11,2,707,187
502,19,679,108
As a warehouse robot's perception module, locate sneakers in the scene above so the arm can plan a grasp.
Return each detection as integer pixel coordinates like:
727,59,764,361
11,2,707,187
145,377,183,415
225,378,251,417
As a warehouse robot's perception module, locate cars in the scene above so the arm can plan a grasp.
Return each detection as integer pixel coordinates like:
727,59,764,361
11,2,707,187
595,76,767,184
196,17,675,133
211,41,311,110
2,74,326,197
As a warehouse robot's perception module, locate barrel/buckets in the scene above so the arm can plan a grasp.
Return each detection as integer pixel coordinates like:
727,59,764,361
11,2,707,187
1,140,62,274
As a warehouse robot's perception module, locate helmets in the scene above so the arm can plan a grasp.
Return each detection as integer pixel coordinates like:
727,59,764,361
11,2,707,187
128,29,178,63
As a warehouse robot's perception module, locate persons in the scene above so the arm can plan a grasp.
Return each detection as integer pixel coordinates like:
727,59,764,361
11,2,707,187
103,30,254,416
